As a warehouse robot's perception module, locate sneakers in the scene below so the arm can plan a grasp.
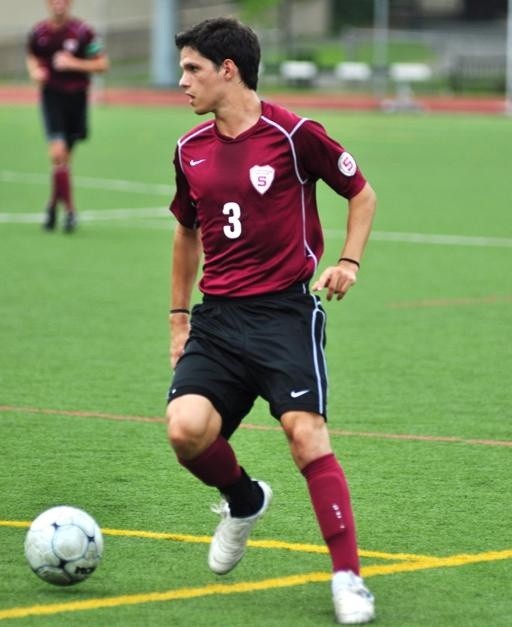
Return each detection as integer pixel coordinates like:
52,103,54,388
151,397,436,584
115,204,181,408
43,209,78,234
207,480,273,575
331,570,376,624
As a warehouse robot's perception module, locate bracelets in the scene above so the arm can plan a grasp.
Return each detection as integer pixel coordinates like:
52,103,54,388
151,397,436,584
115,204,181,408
169,307,190,314
338,258,360,268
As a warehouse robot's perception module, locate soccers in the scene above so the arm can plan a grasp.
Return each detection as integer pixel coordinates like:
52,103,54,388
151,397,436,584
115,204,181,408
24,506,102,584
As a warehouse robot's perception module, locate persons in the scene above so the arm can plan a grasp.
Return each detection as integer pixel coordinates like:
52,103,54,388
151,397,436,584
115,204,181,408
24,0,109,233
162,16,377,625
